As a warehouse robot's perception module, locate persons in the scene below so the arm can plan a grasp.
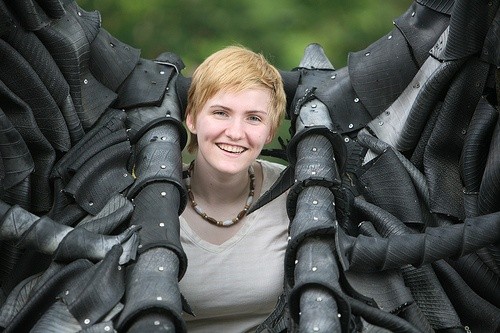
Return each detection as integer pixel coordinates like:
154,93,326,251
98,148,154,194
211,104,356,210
178,45,294,333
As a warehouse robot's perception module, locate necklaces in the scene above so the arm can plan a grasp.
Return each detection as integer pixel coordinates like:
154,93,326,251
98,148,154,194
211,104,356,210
184,160,256,227
